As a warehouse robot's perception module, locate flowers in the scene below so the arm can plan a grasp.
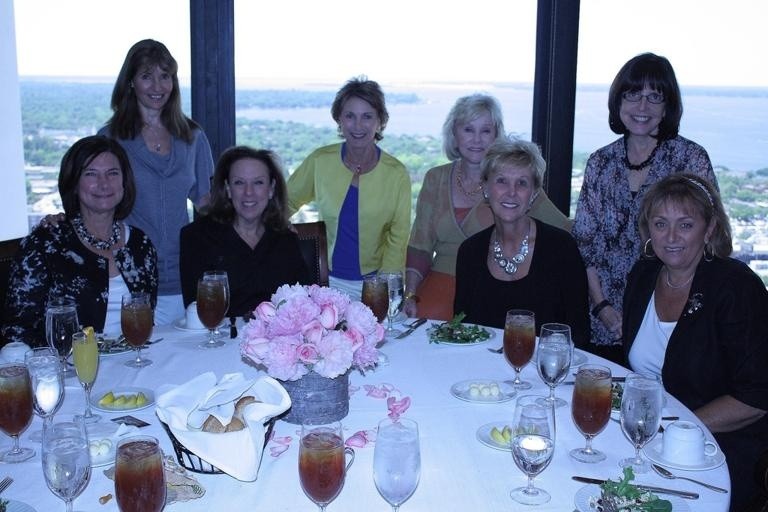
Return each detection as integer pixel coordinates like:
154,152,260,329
240,286,385,382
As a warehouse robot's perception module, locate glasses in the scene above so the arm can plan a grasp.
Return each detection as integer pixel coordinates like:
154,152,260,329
622,88,665,106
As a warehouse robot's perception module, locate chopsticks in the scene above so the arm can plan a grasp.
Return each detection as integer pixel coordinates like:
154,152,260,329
563,372,639,386
661,415,680,420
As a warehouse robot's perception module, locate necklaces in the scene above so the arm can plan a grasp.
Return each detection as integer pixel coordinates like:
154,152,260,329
493,234,530,275
342,149,379,174
623,134,661,172
456,174,486,199
71,213,124,250
666,271,696,289
140,125,173,151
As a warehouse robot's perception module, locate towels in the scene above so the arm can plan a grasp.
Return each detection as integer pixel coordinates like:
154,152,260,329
154,371,292,483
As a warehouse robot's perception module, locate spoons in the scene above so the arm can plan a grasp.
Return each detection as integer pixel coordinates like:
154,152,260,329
650,464,728,494
402,318,421,329
487,346,503,354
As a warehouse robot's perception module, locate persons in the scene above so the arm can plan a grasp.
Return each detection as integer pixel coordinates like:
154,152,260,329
95,38,216,295
405,92,571,322
179,145,313,318
285,72,412,304
453,131,592,350
571,51,722,355
623,172,768,462
2,135,160,349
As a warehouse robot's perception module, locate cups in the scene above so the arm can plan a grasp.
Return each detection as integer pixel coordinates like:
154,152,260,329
185,300,208,329
1,341,33,364
660,420,717,465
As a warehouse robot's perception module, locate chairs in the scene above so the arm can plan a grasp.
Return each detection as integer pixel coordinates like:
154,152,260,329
287,221,329,286
0,237,25,348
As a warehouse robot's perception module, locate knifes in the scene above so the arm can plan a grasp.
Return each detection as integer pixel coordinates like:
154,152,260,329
230,317,237,338
394,318,428,339
572,474,699,502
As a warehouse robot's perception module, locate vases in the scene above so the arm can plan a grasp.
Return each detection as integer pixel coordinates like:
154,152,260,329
276,370,350,424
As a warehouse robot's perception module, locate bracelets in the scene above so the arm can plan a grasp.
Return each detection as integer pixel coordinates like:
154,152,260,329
590,299,614,318
402,290,420,303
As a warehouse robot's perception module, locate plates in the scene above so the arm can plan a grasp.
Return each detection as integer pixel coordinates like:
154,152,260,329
426,324,497,346
86,435,120,468
476,420,551,452
575,477,692,512
641,435,725,472
528,349,589,369
450,377,518,405
172,318,226,332
89,386,157,413
609,383,668,422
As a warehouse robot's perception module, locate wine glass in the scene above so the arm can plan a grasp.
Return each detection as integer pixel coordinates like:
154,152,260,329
298,416,346,512
114,434,167,512
618,370,663,474
372,416,422,512
196,276,228,350
361,277,389,348
24,346,65,451
41,415,93,512
45,299,80,379
121,292,154,369
509,394,555,506
0,363,36,464
382,271,404,337
72,331,100,424
535,323,572,409
202,269,230,339
569,363,612,464
501,308,536,390
